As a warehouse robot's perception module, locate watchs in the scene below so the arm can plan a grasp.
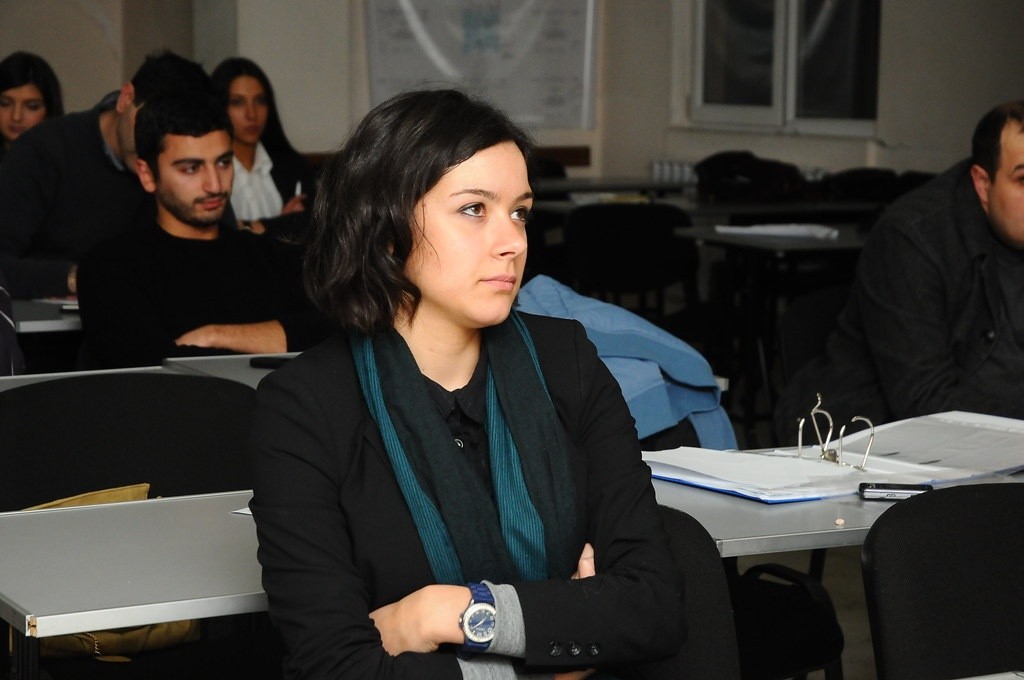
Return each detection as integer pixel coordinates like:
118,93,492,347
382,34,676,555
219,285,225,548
458,582,496,650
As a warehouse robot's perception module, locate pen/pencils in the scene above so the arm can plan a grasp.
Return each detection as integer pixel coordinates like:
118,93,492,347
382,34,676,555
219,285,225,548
296,181,302,196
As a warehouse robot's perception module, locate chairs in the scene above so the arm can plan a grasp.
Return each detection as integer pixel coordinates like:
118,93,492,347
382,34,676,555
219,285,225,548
0,146,1024,679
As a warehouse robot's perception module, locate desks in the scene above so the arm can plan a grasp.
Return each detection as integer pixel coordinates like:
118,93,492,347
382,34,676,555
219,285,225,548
648,469,1023,557
528,179,687,310
0,476,278,680
675,224,866,404
531,200,732,214
1,365,195,395
166,352,310,390
5,295,83,332
707,201,893,222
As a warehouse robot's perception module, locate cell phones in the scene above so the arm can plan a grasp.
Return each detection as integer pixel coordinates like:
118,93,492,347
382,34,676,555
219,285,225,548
858,482,934,501
57,304,80,311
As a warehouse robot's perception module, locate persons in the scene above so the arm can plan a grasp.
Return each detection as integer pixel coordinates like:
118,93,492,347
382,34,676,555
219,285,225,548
0,48,207,298
209,55,308,235
771,101,1023,448
248,87,741,678
77,91,301,498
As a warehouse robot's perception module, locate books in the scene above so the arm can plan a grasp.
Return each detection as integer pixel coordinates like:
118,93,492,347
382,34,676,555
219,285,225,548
640,409,1024,506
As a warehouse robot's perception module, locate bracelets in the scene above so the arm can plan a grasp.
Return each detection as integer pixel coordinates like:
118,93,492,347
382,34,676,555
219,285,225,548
243,221,253,230
